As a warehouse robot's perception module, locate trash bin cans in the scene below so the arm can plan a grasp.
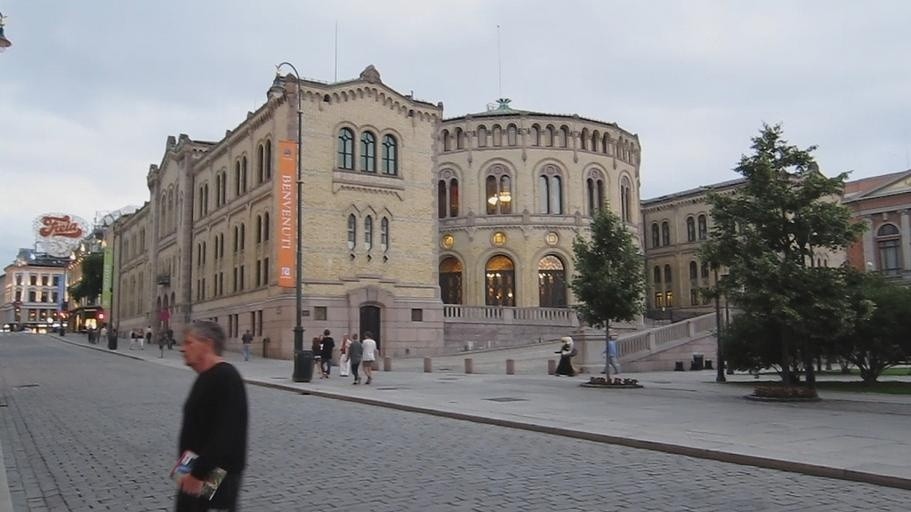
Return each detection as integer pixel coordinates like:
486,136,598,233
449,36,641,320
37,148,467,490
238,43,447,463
292,350,314,382
693,353,704,370
108,336,116,349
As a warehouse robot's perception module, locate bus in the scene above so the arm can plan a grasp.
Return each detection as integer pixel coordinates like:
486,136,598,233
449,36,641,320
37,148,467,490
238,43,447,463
2,321,67,333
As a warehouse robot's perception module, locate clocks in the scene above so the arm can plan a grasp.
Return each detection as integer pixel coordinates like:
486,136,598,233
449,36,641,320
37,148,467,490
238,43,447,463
48,236,69,258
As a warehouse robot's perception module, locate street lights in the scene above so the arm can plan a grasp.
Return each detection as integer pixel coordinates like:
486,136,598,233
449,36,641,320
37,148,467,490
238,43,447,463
708,259,725,383
718,273,735,376
267,60,304,368
101,213,116,327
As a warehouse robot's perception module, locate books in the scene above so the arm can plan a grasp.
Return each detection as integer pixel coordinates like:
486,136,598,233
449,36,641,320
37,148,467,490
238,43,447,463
170,449,227,501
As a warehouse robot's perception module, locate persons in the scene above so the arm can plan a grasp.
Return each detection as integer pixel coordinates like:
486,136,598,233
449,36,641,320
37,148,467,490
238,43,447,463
311,330,377,385
100,327,107,345
555,336,577,377
129,325,173,350
241,330,253,361
601,337,620,375
176,320,249,512
